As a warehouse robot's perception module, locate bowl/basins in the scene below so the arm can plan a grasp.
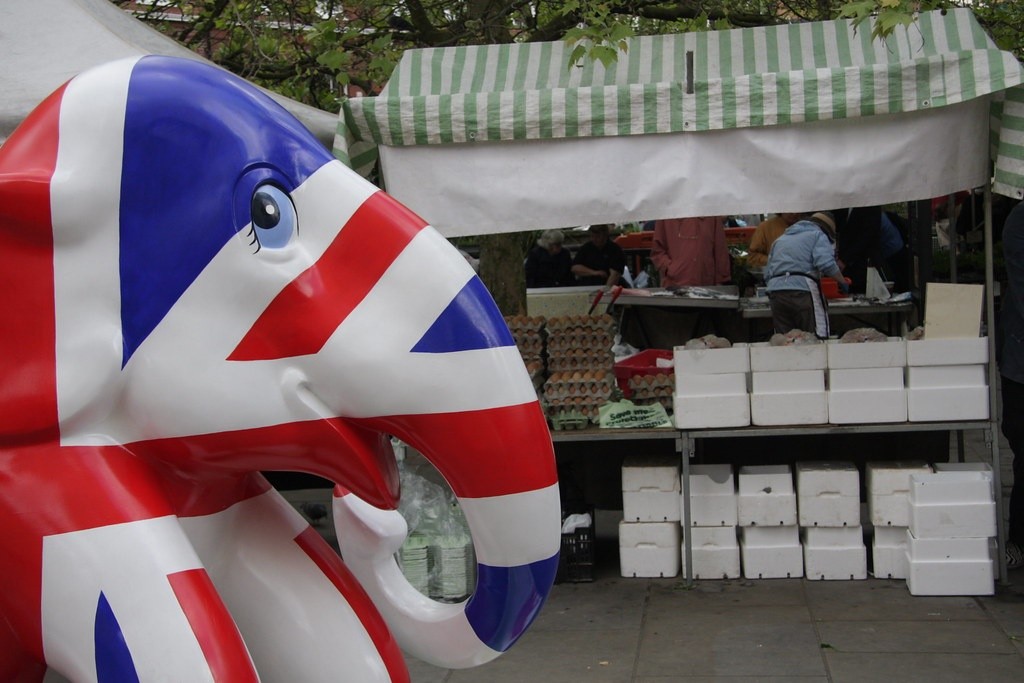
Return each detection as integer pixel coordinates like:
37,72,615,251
819,277,851,299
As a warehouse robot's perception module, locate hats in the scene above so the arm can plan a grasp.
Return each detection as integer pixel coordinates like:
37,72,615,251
811,212,836,235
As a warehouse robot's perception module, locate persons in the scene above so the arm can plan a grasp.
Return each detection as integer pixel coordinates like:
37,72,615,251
999,199,1023,570
521,206,909,340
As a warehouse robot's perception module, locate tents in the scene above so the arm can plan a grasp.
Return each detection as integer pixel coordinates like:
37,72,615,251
331,7,1024,591
0,0,336,147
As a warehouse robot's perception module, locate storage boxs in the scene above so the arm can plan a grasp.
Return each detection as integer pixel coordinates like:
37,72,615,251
553,503,596,583
618,451,996,596
614,348,674,398
672,336,989,430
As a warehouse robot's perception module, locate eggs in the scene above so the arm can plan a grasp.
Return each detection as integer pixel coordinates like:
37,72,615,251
504,312,675,415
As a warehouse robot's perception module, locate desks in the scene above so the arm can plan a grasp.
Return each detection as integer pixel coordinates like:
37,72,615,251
548,421,1010,593
742,293,915,342
622,248,652,279
